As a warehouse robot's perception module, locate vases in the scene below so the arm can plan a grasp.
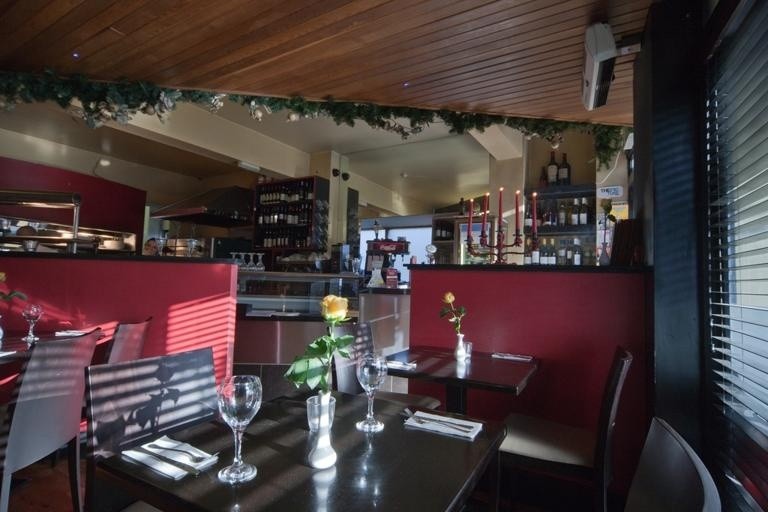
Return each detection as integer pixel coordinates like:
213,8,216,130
599,243,609,266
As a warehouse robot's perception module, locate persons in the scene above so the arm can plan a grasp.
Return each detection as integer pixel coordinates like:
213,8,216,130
143,237,161,257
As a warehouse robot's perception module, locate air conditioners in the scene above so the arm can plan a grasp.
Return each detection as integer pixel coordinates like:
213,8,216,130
578,22,617,112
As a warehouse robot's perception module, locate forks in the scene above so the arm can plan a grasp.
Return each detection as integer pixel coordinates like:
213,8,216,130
148,443,219,463
404,407,470,434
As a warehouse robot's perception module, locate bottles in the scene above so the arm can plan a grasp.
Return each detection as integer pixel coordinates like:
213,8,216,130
524,197,594,232
524,237,594,267
435,221,452,241
539,167,547,186
559,153,571,186
257,203,311,226
260,227,311,248
259,180,313,205
546,151,559,186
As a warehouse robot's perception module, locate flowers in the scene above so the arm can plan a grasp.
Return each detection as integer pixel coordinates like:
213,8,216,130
438,291,467,334
597,199,616,242
283,296,358,397
0,272,27,303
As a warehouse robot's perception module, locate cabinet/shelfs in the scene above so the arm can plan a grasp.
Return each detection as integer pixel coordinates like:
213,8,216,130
251,176,328,254
524,192,595,265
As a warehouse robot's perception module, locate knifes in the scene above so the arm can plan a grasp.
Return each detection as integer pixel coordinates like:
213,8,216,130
133,446,200,478
398,413,472,430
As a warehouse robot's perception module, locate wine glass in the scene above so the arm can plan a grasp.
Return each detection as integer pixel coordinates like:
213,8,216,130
20,304,43,342
256,252,266,271
230,251,237,259
238,253,247,271
247,253,258,271
355,354,387,433
217,374,263,485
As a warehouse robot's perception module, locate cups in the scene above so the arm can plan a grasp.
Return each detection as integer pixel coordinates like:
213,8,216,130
463,342,472,359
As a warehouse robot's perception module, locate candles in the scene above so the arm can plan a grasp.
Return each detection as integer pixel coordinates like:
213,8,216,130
467,187,538,240
